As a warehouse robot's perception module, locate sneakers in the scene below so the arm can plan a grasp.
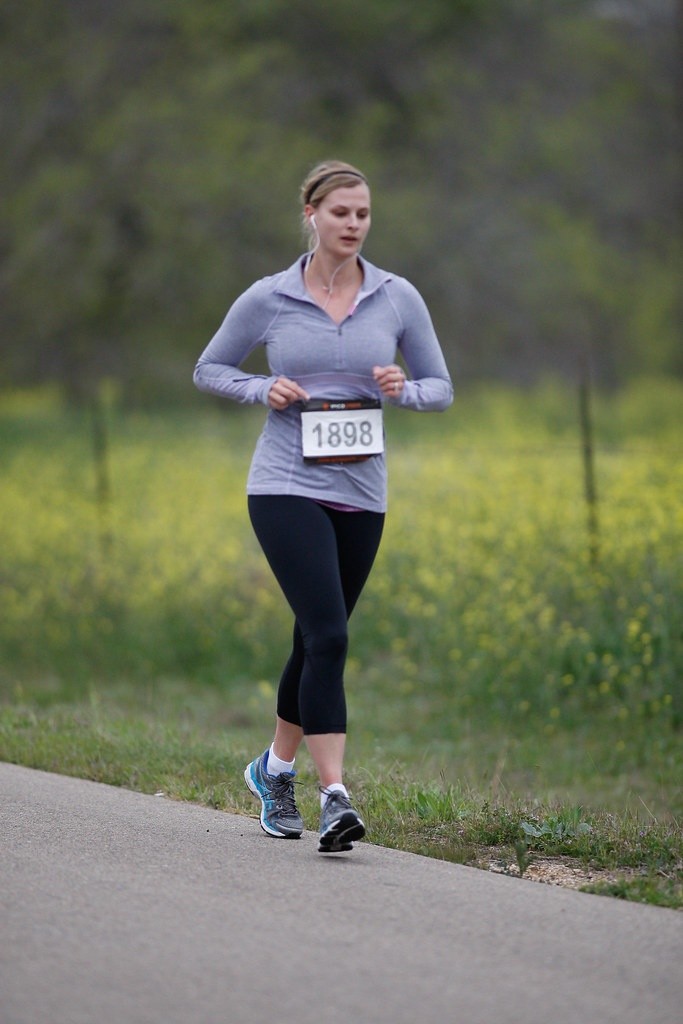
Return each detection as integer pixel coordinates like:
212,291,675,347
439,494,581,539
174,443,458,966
243,750,306,837
315,785,365,852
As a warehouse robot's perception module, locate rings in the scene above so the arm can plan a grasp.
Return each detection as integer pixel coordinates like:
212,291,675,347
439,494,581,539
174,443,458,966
394,382,398,391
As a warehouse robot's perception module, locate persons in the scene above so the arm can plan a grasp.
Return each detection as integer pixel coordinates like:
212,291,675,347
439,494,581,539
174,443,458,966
192,158,453,852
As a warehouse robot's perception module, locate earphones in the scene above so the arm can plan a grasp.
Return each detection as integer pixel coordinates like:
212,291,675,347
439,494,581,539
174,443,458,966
310,215,316,229
